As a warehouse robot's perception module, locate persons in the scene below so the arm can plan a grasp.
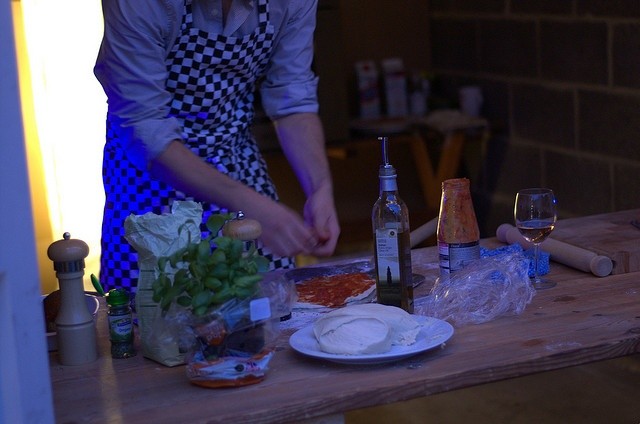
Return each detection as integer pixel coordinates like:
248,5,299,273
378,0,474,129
94,1,341,295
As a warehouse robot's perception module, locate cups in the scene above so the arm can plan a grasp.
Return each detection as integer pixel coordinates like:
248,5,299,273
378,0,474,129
459,87,486,120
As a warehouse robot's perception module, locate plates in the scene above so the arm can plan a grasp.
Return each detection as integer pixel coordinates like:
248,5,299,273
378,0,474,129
40,293,100,352
289,315,454,366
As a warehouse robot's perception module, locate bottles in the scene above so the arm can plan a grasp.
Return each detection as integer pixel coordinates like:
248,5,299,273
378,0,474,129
46,232,99,366
370,136,416,314
105,288,138,359
222,210,263,259
436,178,482,290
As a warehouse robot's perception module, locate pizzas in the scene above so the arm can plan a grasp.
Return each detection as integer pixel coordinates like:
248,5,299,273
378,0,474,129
295,271,376,308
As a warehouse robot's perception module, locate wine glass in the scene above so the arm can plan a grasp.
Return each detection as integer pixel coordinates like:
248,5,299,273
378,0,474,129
513,188,559,290
